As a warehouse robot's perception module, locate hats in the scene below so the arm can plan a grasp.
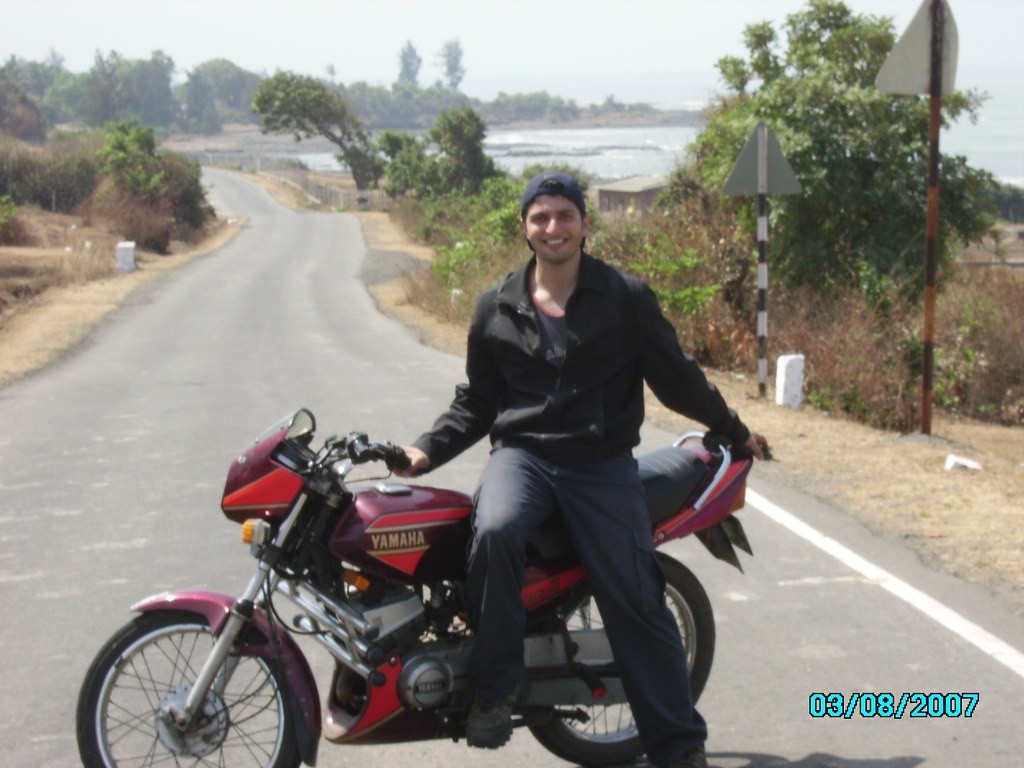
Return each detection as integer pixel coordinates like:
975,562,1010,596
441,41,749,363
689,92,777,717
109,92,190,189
522,172,586,216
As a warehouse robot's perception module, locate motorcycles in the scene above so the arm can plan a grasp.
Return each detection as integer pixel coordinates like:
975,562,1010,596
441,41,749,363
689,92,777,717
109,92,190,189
76,405,754,768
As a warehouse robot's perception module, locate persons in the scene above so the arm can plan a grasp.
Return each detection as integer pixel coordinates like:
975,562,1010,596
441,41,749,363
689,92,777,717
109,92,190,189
388,172,773,768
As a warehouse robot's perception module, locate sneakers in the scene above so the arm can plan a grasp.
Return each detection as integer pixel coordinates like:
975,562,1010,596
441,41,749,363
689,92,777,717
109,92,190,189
466,677,531,750
667,742,708,768
